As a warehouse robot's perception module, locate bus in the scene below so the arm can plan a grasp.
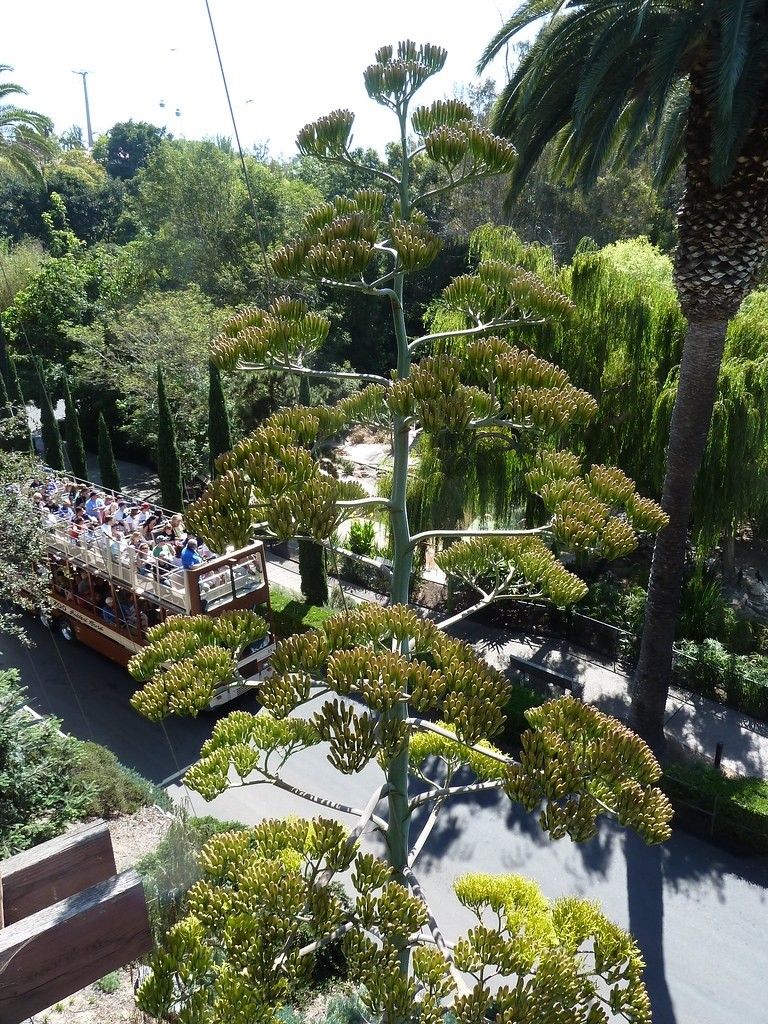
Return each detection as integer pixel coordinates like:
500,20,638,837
2,453,279,713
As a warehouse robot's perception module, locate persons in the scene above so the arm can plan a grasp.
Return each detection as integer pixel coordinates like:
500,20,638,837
24,469,265,599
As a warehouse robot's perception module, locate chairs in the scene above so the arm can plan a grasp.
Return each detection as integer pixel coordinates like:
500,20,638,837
25,462,257,636
